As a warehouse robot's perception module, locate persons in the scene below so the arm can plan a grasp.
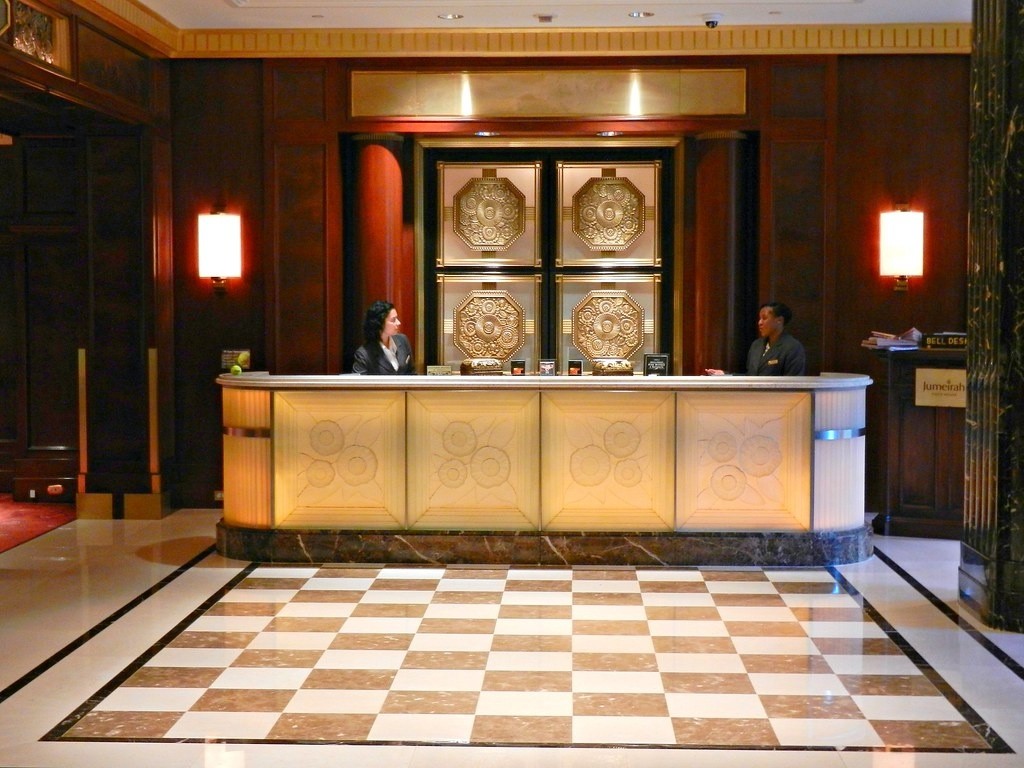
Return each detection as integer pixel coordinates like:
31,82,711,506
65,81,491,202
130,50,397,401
705,302,806,377
351,300,418,375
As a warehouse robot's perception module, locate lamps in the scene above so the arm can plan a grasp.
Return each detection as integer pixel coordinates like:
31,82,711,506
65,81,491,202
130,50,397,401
198,199,241,296
879,198,924,298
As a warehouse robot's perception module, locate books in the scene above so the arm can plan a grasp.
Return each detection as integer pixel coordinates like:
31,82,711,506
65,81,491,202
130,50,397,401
861,328,923,352
